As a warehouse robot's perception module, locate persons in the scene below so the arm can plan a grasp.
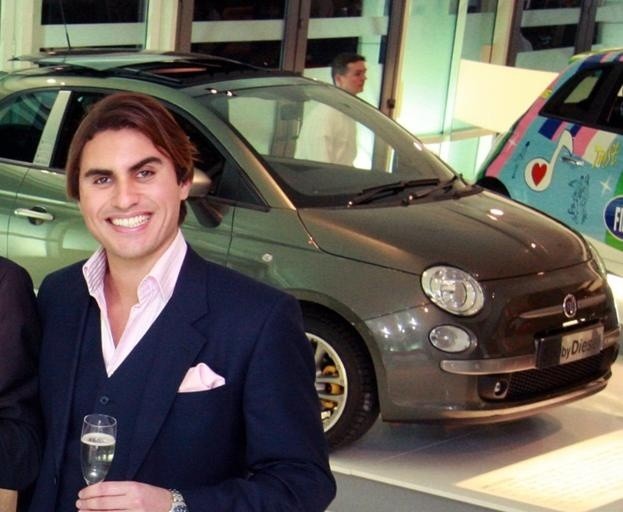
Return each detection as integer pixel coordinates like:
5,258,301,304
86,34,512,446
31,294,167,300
304,52,368,168
27,92,336,512
0,256,36,512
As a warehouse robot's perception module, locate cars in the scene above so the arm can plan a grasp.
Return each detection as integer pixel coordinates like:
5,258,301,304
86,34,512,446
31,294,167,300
476,48,622,273
0,43,623,456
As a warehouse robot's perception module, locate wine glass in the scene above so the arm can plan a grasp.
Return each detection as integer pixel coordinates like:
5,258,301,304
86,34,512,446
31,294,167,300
78,413,117,487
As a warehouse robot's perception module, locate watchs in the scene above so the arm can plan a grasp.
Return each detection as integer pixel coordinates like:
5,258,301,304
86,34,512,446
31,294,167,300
168,488,189,512
